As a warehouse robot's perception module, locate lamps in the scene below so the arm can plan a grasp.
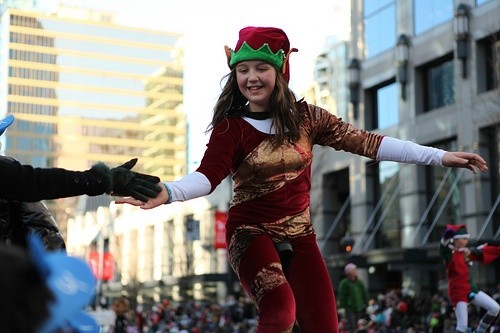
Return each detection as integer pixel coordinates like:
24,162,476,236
391,34,412,104
456,4,473,80
345,58,365,121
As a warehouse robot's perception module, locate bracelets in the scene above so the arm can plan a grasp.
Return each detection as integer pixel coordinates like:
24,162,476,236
162,182,173,205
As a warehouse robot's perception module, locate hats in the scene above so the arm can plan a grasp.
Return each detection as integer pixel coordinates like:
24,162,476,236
0,114,15,137
223,26,298,84
446,224,471,240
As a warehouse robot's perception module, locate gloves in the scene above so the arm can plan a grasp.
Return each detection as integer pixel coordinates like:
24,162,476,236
444,229,457,241
95,158,163,203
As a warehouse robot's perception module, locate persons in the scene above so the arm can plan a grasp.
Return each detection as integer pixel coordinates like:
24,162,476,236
0,115,163,333
336,262,370,333
438,223,500,333
113,281,260,333
333,288,500,333
114,27,489,333
